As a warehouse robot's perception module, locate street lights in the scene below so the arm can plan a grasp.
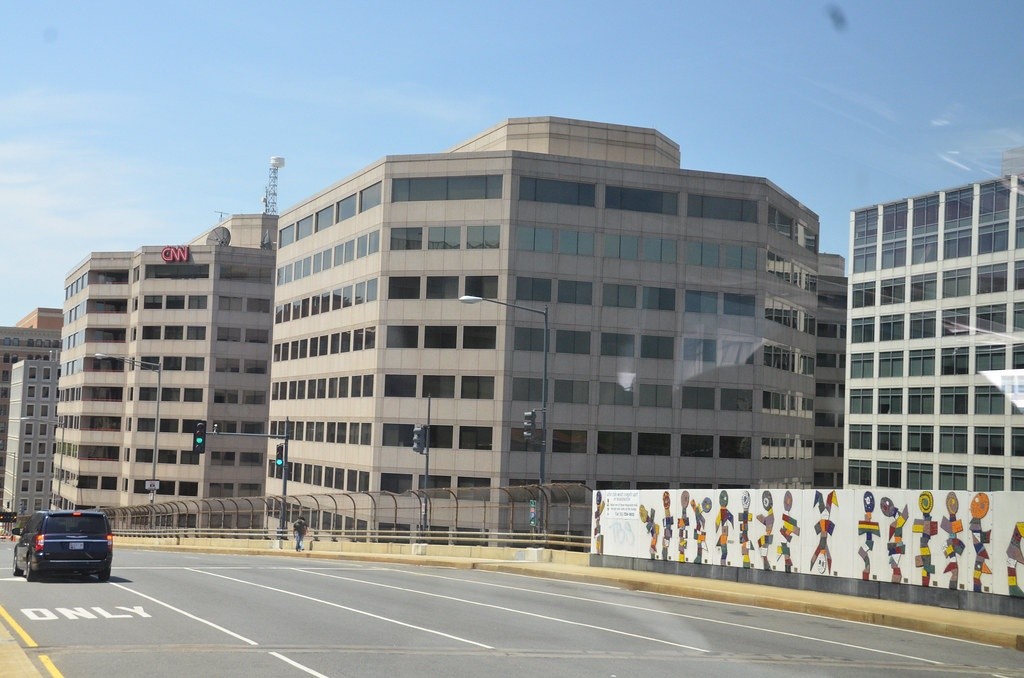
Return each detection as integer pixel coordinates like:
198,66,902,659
94,352,164,506
456,294,551,535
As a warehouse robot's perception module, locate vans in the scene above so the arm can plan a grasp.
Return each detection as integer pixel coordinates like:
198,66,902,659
11,507,114,582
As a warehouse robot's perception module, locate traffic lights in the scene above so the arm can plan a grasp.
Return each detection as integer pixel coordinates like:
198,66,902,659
413,427,420,452
522,411,532,438
275,444,283,468
1,512,17,522
195,423,204,452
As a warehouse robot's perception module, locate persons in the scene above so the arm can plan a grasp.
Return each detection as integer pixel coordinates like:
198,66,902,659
293,516,307,552
